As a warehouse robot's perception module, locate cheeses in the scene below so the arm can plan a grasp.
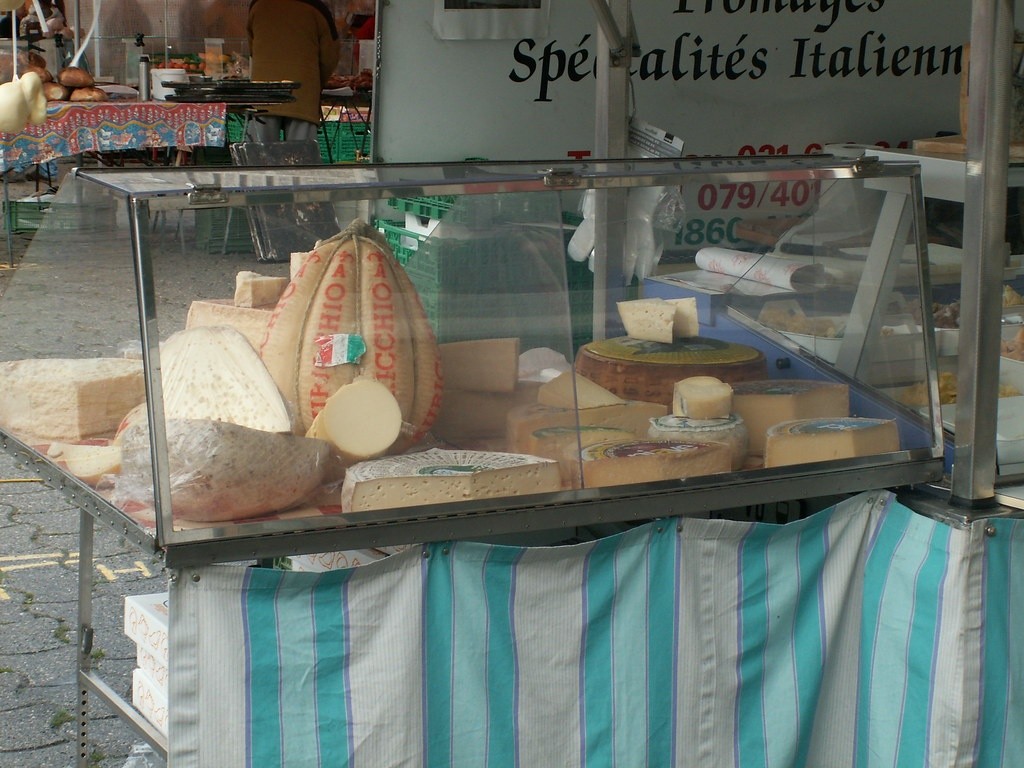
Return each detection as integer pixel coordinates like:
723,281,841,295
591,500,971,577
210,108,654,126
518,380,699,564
0,271,901,518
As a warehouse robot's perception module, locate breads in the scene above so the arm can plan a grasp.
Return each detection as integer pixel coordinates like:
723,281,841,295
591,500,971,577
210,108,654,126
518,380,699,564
22,66,109,102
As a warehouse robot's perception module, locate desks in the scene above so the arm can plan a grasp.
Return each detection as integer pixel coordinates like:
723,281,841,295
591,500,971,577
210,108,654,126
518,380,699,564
0,97,226,268
319,94,372,163
173,102,282,166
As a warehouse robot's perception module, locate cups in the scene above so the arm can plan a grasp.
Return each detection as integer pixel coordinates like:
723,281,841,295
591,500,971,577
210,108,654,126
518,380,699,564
138,57,150,102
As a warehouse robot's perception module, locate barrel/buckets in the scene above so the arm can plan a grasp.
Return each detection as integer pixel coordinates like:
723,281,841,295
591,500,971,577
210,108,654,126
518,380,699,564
150,69,186,100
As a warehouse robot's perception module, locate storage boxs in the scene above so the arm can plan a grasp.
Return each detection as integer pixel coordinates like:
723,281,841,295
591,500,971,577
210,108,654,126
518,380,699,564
373,156,594,364
192,112,371,255
3,199,118,233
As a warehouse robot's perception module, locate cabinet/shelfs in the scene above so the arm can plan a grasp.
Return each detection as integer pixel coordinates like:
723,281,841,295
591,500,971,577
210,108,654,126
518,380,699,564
0,147,1024,768
